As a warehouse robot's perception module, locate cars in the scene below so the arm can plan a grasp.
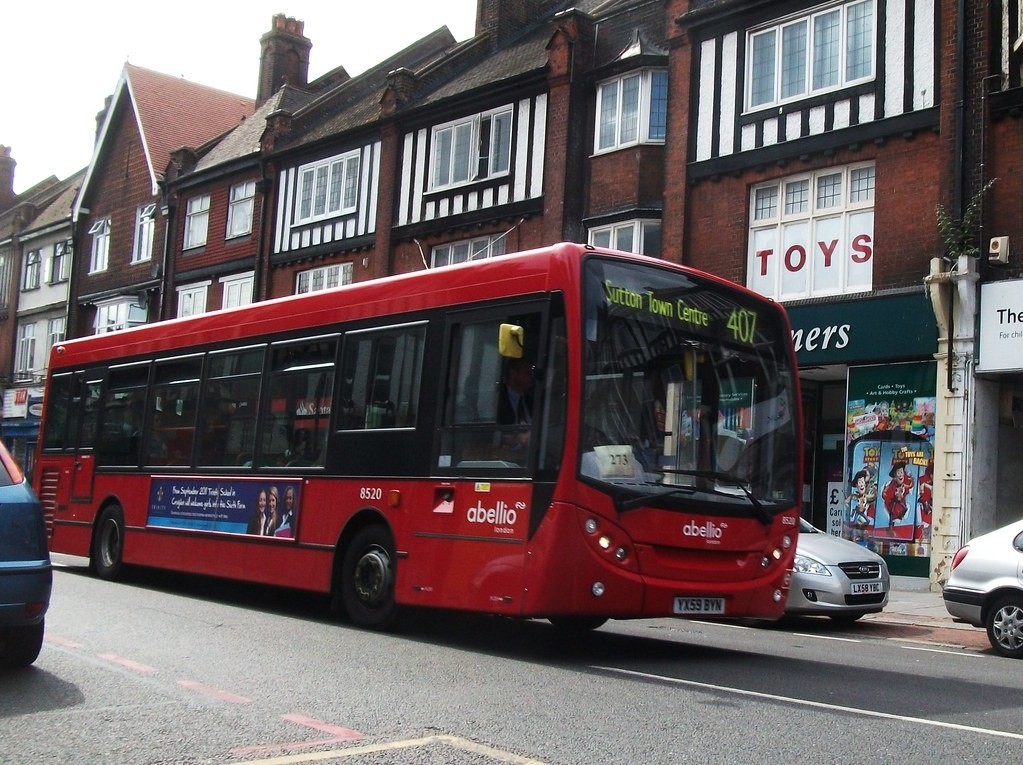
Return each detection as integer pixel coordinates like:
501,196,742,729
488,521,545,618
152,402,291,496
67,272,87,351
1,438,52,667
943,519,1023,656
737,514,891,626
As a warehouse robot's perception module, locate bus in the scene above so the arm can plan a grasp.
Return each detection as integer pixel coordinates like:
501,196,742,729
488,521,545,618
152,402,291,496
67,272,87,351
34,241,805,631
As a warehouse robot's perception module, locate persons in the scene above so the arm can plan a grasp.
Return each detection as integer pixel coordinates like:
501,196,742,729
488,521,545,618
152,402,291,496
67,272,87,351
245,485,299,537
482,357,534,460
74,381,321,466
